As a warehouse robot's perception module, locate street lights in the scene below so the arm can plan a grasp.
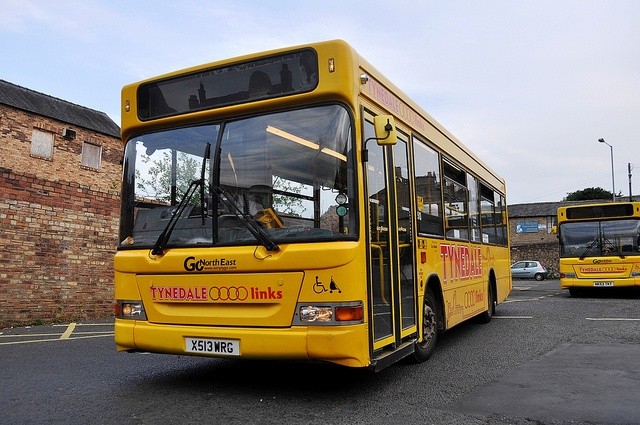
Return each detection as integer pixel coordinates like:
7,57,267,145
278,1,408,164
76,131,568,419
598,137,615,203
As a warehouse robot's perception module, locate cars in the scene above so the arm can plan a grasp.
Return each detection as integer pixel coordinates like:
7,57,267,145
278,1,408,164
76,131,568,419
511,261,547,280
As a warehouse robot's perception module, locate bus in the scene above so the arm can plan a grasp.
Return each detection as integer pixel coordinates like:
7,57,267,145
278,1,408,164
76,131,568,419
113,38,512,373
552,202,640,298
